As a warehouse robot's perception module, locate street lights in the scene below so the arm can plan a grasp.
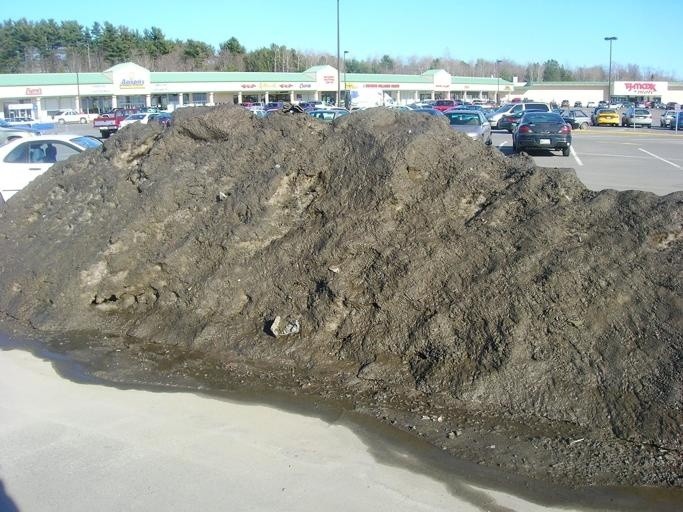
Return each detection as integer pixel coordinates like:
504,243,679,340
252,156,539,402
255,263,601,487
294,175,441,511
342,49,350,101
603,35,617,99
495,58,502,104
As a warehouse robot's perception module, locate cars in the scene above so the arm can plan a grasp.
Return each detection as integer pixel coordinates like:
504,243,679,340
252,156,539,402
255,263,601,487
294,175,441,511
51,108,88,125
0,134,103,202
510,110,573,159
0,113,55,147
